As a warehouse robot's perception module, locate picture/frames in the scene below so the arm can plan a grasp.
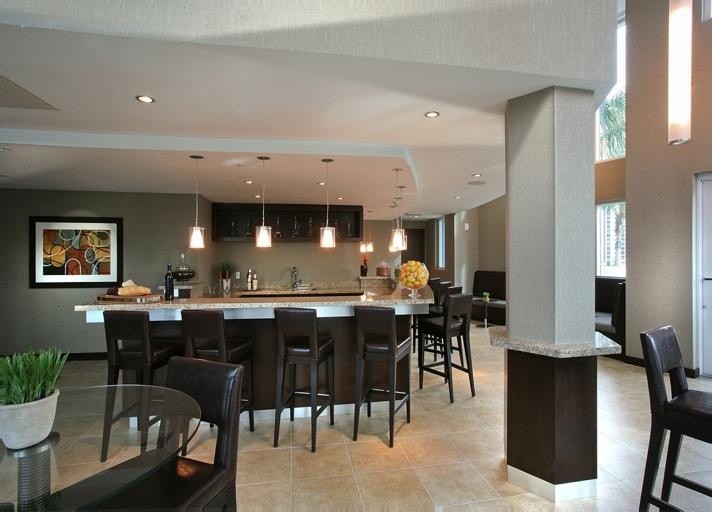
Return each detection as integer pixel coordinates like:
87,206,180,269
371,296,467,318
28,215,125,290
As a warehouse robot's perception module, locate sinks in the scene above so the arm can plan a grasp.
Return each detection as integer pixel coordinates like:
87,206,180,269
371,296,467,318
230,288,365,299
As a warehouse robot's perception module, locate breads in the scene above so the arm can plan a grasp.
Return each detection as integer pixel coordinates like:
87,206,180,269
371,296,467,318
118,285,152,296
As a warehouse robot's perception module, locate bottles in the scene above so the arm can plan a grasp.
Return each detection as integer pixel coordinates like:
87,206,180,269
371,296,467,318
246,268,258,291
164,263,174,301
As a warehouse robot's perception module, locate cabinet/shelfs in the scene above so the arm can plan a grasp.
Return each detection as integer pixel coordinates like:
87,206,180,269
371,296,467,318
210,201,364,244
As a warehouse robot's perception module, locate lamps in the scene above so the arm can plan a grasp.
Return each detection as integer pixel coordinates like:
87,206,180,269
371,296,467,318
255,158,273,248
359,209,374,254
187,157,206,249
318,161,337,249
387,167,408,253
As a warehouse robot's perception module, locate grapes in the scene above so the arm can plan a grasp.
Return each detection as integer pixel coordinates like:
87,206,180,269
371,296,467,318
106,286,118,295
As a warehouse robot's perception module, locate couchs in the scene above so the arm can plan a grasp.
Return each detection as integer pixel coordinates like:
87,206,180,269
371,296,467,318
471,270,626,359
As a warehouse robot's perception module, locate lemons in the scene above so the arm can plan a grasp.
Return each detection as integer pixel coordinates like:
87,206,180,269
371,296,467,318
398,260,429,288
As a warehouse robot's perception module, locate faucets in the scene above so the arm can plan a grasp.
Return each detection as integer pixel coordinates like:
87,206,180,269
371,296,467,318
288,264,301,288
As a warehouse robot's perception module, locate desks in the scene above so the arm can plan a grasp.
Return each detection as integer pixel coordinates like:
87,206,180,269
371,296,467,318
1,384,202,512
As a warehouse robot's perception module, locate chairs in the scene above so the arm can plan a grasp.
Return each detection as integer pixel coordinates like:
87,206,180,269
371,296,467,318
636,321,711,511
89,352,244,512
351,305,411,449
100,309,172,462
180,308,255,434
273,307,335,453
413,276,476,404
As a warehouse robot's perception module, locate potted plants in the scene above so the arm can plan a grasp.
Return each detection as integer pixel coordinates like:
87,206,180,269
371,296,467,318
1,345,72,448
